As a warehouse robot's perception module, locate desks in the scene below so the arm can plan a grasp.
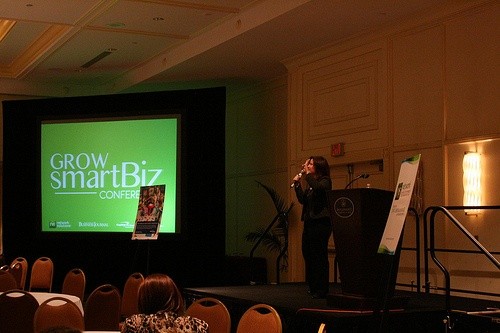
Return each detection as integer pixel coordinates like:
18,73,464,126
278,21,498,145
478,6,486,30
0,291,84,318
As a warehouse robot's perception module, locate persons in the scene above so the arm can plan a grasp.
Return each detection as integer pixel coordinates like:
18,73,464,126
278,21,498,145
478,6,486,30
120,274,209,333
293,156,332,299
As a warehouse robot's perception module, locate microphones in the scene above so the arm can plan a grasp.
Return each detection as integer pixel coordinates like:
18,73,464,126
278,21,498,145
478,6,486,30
290,170,305,188
345,173,369,189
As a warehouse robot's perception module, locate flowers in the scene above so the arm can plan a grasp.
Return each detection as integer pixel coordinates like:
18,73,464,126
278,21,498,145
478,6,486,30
119,312,208,333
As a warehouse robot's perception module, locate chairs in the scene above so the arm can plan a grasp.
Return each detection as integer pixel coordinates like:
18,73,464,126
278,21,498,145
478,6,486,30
62,269,86,307
84,285,120,330
122,273,144,319
185,298,230,333
34,297,83,333
0,257,28,291
29,257,53,293
238,304,282,333
0,288,40,333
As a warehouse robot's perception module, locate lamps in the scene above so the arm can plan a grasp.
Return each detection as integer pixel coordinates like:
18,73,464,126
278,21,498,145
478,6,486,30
462,151,483,216
